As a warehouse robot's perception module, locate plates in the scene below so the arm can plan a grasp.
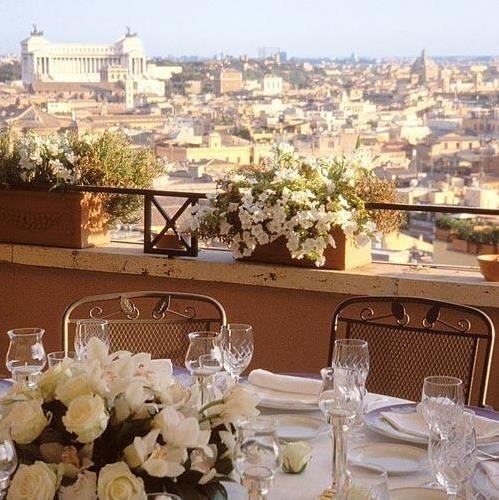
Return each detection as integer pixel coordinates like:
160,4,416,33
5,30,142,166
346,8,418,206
255,391,499,500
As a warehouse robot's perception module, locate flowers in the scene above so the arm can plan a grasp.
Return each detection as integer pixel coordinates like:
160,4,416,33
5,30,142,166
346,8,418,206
180,134,409,266
0,337,314,500
1,119,165,225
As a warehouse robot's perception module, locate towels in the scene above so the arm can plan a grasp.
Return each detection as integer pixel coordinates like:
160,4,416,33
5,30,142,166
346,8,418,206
379,408,499,439
247,368,324,395
477,455,499,490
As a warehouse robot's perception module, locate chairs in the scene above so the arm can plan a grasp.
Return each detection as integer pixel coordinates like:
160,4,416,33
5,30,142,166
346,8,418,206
63,290,228,368
327,296,495,409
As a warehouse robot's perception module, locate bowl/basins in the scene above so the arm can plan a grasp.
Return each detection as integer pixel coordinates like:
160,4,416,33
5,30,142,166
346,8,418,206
153,232,189,250
478,254,498,282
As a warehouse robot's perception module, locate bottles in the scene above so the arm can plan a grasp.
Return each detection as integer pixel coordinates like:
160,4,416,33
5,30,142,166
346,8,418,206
422,376,463,489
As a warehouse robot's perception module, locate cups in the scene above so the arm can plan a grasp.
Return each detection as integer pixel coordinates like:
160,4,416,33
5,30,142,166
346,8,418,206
0,428,18,496
8,319,120,379
238,418,283,500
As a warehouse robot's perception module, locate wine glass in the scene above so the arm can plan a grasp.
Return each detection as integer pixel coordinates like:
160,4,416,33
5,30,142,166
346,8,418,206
184,325,253,407
320,338,370,499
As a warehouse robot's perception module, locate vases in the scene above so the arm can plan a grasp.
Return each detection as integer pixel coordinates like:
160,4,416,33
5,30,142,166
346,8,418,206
476,254,499,283
153,230,191,248
237,226,374,271
2,192,111,247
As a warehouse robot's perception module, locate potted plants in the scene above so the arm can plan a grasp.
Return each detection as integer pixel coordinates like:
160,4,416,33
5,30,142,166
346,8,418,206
434,216,499,256
